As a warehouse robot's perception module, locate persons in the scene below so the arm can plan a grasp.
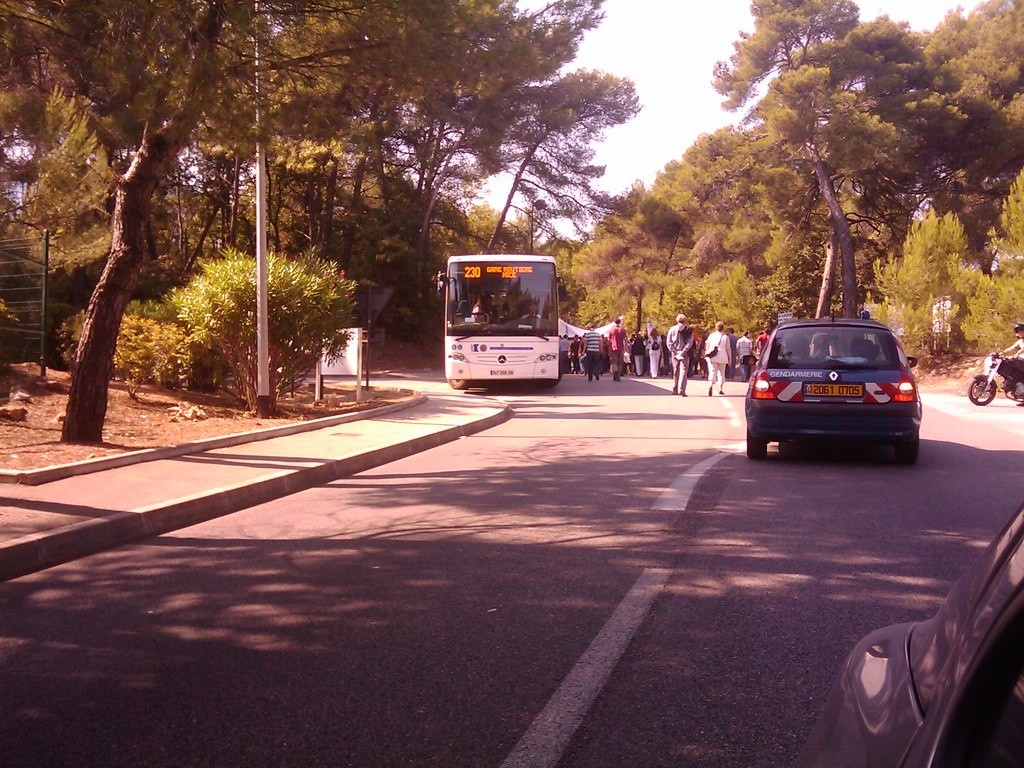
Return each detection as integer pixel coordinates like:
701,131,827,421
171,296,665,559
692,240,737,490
666,313,694,397
558,319,770,381
581,323,603,381
470,298,487,320
990,324,1024,393
608,318,628,382
520,303,542,321
784,334,809,361
704,321,732,397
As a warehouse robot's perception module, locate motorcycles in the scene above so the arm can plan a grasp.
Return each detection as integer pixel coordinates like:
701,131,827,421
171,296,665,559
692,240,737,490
967,343,1024,407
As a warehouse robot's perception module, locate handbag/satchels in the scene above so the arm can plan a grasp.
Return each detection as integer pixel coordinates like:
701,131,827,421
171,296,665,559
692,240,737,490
704,346,719,358
660,352,665,368
623,351,631,364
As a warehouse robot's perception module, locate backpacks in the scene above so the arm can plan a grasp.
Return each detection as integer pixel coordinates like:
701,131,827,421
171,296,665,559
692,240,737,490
651,341,660,350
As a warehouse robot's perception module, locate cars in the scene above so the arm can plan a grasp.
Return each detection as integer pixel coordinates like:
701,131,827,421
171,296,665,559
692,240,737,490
740,314,923,466
786,498,1024,768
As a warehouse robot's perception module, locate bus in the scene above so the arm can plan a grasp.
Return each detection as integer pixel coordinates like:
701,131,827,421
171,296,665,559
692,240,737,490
437,253,567,390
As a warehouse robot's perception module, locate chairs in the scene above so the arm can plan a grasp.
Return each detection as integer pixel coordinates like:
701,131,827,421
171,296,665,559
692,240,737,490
784,334,810,357
456,300,471,316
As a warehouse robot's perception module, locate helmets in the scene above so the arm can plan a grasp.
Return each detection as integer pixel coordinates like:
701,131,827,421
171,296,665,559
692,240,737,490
1014,323,1024,333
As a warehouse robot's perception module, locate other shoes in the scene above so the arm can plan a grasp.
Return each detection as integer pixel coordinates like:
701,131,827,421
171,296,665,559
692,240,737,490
719,391,724,394
708,387,712,396
1015,386,1024,395
596,376,599,381
682,394,687,397
637,375,640,377
